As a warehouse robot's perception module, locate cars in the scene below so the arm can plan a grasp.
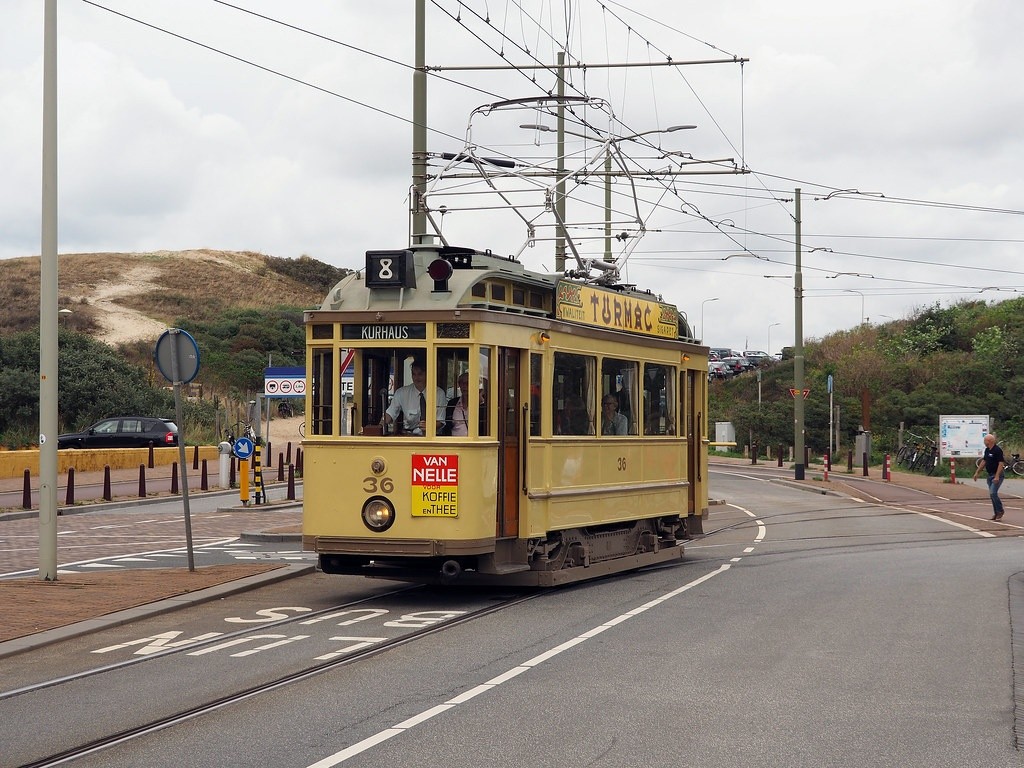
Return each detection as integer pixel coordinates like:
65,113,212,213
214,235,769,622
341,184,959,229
707,363,734,383
732,350,742,357
743,350,770,364
722,357,755,374
708,351,721,362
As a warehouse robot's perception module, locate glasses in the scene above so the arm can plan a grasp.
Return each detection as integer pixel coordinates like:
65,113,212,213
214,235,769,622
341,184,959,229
603,403,614,406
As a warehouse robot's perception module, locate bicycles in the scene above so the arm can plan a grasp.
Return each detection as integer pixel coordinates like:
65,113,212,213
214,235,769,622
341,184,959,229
976,439,1024,479
224,424,239,445
895,429,925,470
298,412,314,439
237,418,255,439
912,436,941,475
280,401,294,419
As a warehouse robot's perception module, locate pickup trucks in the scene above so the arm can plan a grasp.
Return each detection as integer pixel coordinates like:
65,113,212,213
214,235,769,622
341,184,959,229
771,352,783,360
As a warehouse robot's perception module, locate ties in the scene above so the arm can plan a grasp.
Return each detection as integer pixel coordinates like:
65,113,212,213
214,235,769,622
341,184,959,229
419,393,426,420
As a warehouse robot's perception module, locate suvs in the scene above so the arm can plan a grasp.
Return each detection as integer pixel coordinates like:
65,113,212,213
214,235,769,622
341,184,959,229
58,417,178,449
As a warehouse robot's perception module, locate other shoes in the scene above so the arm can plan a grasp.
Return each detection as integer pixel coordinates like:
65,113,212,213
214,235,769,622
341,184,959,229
991,513,996,520
996,509,1005,519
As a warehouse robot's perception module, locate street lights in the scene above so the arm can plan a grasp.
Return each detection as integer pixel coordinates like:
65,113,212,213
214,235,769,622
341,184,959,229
701,297,720,345
842,289,865,326
767,323,780,355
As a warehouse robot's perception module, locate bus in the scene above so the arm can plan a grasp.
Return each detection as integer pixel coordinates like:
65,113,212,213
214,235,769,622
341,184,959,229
297,238,711,588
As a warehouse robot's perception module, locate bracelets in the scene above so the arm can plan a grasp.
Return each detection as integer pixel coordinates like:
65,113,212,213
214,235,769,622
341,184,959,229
379,423,383,427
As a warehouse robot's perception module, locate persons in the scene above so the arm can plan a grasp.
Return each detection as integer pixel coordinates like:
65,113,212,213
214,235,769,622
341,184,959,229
446,374,487,437
973,434,1005,521
601,395,628,435
379,358,448,437
560,392,595,435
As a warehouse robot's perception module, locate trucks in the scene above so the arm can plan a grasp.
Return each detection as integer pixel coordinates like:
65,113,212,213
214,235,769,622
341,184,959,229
710,347,731,357
